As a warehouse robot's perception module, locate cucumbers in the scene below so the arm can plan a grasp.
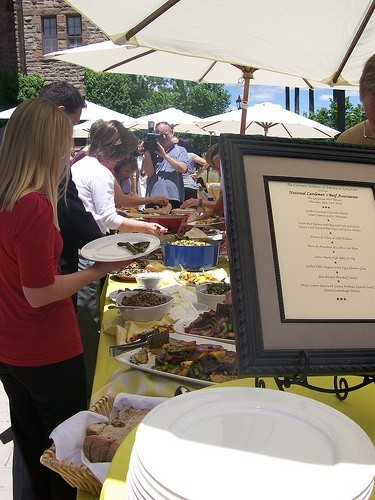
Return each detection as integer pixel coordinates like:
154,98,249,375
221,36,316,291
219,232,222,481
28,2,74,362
186,363,209,381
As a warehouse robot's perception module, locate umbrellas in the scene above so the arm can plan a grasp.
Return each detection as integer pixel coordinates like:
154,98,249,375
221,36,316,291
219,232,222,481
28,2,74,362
41,38,360,135
190,102,341,141
0,100,139,131
67,0,375,89
85,106,220,136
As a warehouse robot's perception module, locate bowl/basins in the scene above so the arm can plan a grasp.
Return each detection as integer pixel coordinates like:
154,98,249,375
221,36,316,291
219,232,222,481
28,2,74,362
117,291,174,322
135,273,162,287
193,303,209,311
142,216,189,235
196,282,231,309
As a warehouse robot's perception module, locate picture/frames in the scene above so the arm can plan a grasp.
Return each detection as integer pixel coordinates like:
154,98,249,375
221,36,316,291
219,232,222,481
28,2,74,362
218,133,375,379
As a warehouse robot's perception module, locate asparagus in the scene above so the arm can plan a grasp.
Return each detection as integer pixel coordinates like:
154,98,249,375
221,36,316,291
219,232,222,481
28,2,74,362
117,242,145,254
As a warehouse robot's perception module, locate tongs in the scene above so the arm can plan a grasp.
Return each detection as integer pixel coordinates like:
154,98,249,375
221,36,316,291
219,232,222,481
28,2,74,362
108,326,168,357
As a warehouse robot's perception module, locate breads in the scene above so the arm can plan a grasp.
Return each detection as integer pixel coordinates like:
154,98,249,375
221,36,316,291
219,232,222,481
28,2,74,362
82,405,150,463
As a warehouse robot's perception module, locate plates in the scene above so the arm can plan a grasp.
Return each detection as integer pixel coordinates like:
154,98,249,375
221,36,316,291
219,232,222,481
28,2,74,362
115,332,237,386
114,274,136,281
81,233,161,262
119,210,154,216
110,287,173,302
187,217,225,227
174,311,235,344
125,386,375,500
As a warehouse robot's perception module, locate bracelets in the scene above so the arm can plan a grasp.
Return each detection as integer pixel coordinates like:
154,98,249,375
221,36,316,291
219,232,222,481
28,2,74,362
198,198,202,206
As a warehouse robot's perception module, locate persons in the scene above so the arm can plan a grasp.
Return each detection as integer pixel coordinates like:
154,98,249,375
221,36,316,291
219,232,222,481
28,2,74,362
36,81,108,316
335,54,375,146
0,98,137,500
70,118,229,326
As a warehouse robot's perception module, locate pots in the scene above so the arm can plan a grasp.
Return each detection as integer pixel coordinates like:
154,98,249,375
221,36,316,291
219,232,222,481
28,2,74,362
161,239,226,271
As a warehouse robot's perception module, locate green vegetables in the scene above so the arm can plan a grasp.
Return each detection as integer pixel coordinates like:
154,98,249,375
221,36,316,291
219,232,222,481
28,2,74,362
205,278,231,296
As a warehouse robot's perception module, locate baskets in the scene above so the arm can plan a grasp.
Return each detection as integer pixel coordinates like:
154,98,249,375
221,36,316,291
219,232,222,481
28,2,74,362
37,392,116,496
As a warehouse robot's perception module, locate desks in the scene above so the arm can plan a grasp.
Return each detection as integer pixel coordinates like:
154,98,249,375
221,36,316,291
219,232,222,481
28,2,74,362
88,211,375,500
